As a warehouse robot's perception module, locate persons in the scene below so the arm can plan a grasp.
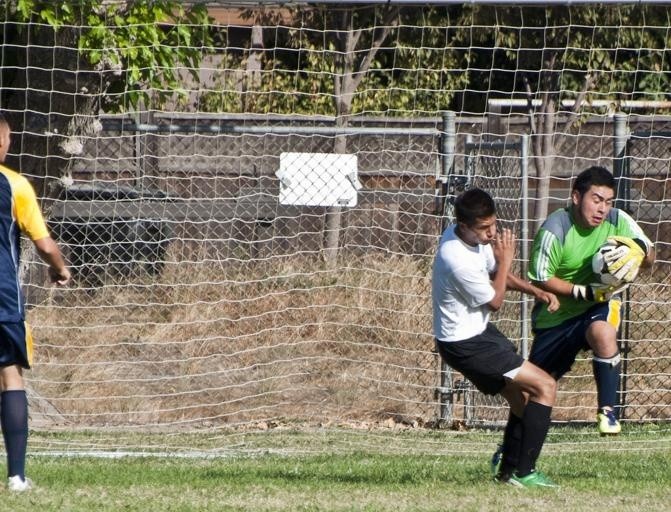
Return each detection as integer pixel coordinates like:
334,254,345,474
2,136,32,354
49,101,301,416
491,166,656,472
432,189,561,489
0,113,70,491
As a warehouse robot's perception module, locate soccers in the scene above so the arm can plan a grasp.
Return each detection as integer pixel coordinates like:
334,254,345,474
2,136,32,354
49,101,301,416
592,243,639,288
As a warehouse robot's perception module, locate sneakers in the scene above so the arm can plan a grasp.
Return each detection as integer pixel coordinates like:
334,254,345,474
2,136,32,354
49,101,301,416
7,475,32,492
491,442,559,491
594,409,621,434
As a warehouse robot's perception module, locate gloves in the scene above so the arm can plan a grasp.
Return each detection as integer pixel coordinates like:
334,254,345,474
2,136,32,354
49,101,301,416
603,236,651,283
572,284,630,303
